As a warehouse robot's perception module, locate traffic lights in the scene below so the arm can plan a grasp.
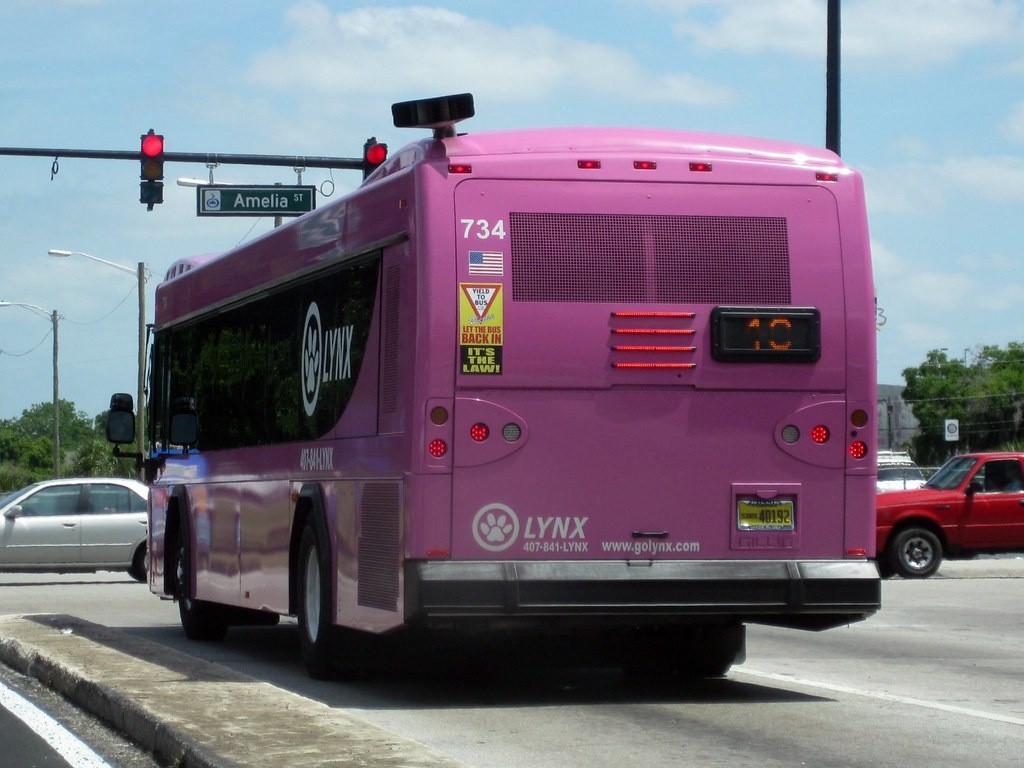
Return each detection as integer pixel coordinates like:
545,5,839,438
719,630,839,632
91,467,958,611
139,134,164,204
361,142,387,179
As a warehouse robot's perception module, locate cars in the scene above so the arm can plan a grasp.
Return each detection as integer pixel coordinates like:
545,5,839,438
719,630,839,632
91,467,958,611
1,477,153,583
878,452,1024,579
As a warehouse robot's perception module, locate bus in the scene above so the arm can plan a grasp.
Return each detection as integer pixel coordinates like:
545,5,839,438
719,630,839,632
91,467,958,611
104,92,881,675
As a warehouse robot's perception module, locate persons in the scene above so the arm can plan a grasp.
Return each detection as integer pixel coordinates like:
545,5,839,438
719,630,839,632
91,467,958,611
986,463,1022,493
